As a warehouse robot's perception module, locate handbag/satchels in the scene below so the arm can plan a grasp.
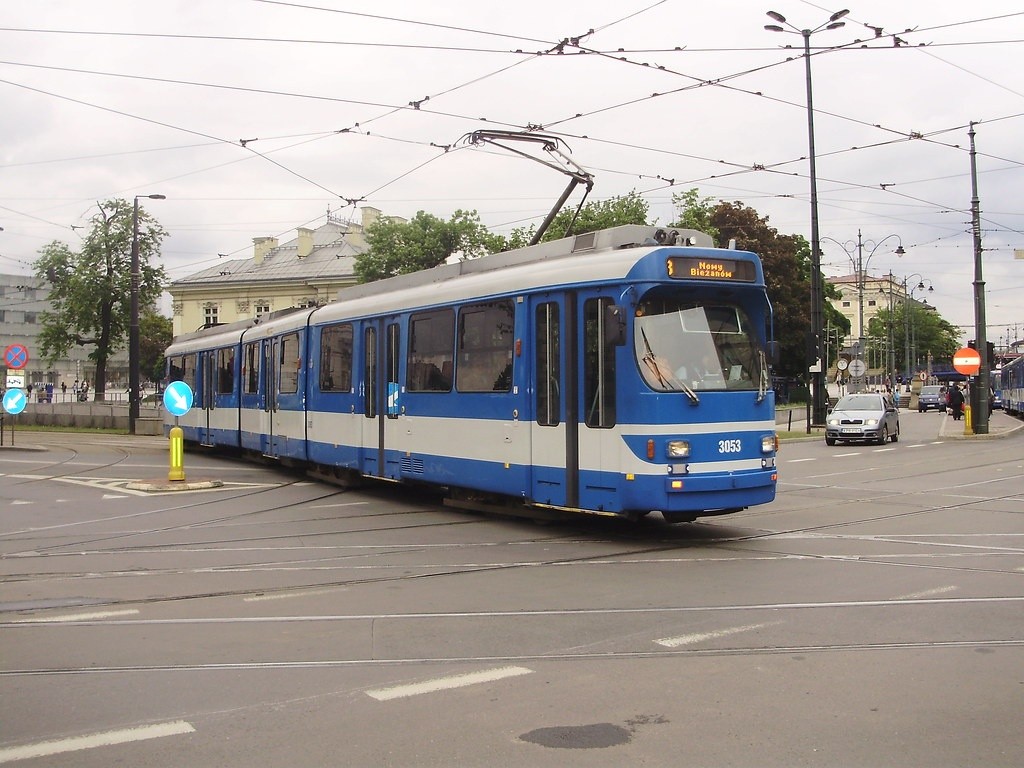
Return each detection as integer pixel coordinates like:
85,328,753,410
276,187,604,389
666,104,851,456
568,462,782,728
962,403,965,411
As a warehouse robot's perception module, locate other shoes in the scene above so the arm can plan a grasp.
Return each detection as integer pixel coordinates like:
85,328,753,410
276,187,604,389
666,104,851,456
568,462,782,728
954,418,956,420
958,418,960,420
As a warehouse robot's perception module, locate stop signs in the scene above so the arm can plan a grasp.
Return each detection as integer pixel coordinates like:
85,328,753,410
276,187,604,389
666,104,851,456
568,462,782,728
953,348,981,375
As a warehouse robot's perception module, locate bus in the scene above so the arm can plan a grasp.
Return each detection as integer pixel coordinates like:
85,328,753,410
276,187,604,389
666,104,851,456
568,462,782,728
160,224,780,525
1001,353,1024,419
989,371,1004,409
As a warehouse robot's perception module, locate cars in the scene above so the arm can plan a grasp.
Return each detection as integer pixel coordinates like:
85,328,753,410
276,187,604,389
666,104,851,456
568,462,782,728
824,393,900,447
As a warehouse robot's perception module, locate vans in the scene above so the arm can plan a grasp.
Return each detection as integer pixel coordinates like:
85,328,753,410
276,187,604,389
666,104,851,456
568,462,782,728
916,384,945,412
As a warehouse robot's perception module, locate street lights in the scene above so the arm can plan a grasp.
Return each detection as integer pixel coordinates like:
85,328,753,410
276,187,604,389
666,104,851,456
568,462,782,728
130,193,166,431
820,228,906,394
879,270,934,401
763,8,850,430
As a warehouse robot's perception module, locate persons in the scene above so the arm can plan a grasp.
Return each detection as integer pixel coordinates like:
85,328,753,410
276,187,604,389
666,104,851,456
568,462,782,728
836,372,845,387
886,372,903,409
26,385,32,398
937,382,970,420
139,385,145,406
824,377,828,388
61,379,91,401
988,379,995,421
